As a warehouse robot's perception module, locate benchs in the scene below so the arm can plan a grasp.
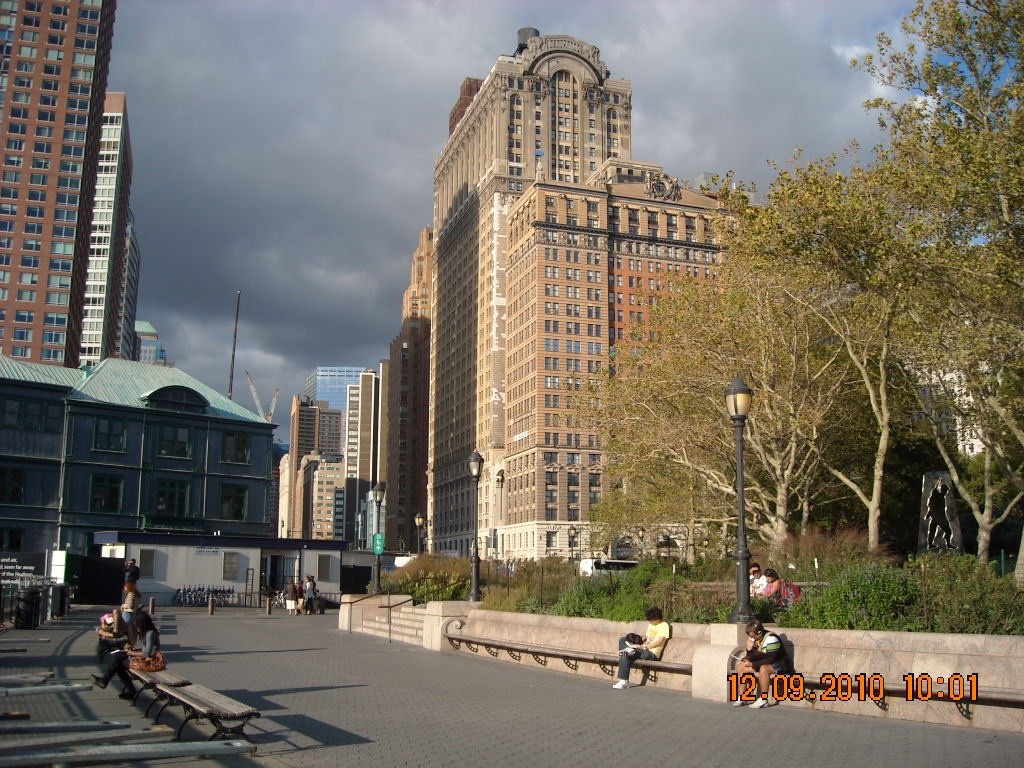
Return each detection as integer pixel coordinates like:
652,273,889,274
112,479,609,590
443,618,692,682
752,671,1024,720
99,634,260,759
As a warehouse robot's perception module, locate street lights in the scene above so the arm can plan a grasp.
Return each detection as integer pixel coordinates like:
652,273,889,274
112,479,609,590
638,526,646,558
723,376,761,624
467,449,485,603
567,524,577,567
414,512,424,555
371,483,387,596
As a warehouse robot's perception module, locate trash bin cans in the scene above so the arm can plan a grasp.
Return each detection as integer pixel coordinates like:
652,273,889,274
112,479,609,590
52,585,66,617
15,587,41,629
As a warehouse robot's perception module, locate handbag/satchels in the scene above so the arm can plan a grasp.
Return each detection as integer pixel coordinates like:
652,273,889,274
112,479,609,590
130,649,166,672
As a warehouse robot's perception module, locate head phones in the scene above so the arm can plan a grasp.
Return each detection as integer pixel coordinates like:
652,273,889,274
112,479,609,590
748,622,763,637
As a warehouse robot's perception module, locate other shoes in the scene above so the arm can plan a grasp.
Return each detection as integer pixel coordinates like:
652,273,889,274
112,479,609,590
88,674,106,689
118,688,134,698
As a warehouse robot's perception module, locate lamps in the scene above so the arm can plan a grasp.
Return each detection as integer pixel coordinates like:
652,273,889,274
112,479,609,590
143,463,156,473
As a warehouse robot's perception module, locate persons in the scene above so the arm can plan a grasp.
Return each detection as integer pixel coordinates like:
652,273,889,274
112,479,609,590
733,620,794,709
748,563,787,608
90,581,161,700
613,608,669,689
121,559,140,604
286,576,316,616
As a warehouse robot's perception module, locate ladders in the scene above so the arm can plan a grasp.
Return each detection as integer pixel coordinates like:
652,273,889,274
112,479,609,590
244,568,254,607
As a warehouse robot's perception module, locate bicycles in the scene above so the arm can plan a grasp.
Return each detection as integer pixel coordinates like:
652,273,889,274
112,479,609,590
176,584,239,607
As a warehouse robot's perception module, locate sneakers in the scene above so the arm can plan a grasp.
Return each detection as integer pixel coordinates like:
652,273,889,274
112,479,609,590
748,698,768,708
613,680,629,689
618,648,635,656
733,696,748,706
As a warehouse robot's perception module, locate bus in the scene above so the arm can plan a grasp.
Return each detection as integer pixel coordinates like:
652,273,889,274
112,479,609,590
580,559,639,577
